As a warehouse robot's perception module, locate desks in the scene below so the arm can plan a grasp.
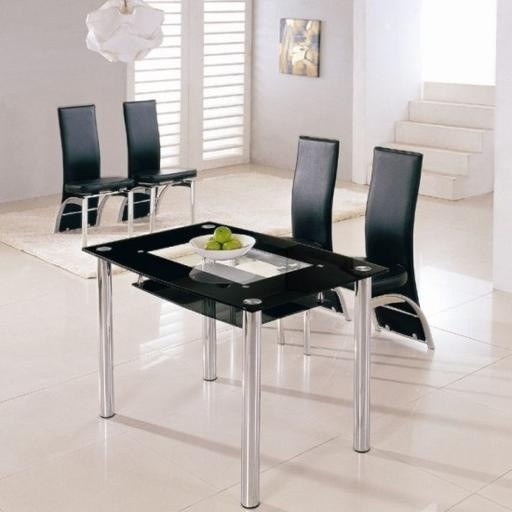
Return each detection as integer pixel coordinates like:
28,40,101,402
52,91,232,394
81,221,389,510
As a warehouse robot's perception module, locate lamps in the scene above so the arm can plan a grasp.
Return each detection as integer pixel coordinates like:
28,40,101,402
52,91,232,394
85,0,167,67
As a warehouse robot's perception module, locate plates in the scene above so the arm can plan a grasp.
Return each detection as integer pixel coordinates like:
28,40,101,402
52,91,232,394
189,262,256,284
189,233,257,259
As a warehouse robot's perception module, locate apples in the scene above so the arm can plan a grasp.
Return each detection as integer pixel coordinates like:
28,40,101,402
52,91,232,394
205,225,241,251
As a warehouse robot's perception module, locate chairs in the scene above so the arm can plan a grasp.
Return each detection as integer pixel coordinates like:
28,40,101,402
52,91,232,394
272,136,353,354
117,100,199,235
320,146,437,352
52,104,137,247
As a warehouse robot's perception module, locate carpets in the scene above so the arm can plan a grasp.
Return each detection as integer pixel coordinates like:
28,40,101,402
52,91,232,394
1,170,369,278
